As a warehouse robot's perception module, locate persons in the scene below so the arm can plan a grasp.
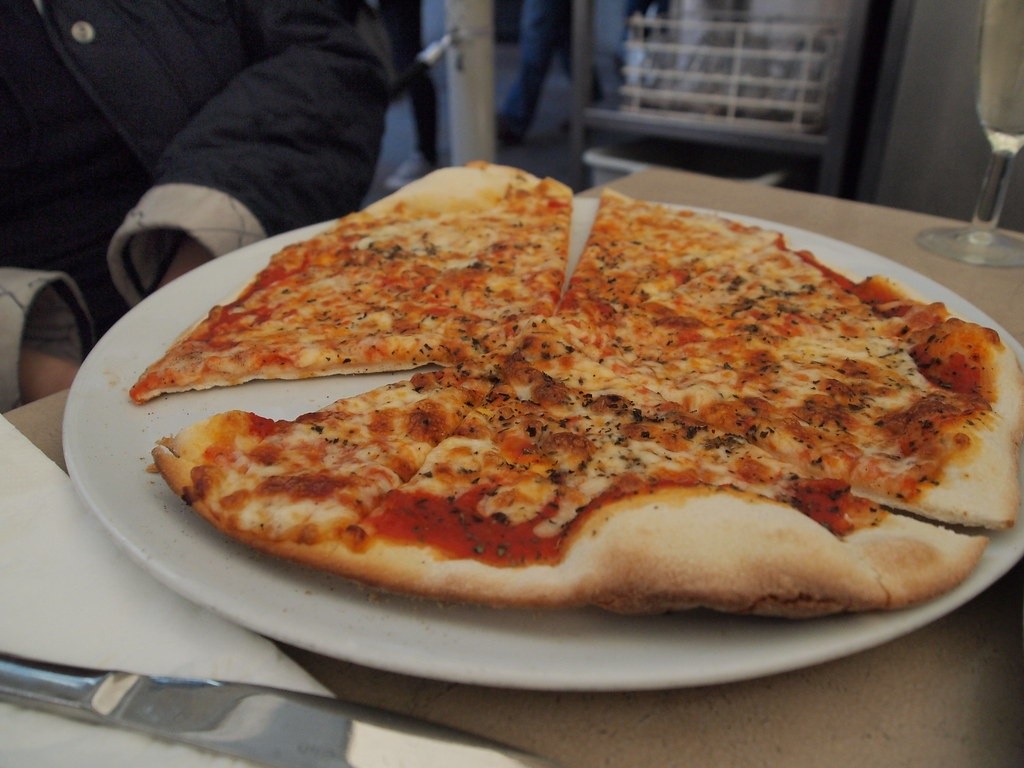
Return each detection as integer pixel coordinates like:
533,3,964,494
386,0,607,195
0,0,396,415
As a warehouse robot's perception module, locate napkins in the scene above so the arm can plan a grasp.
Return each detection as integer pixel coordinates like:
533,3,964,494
1,416,340,767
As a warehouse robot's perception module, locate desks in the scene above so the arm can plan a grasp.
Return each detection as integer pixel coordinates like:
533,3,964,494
1,164,1023,768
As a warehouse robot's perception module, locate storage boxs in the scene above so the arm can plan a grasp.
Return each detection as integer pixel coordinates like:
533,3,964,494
583,135,791,192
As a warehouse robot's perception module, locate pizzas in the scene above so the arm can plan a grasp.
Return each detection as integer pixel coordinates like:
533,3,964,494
152,346,989,621
504,188,1023,530
128,161,574,404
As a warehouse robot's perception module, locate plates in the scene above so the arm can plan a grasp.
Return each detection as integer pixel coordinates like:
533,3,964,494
61,199,1024,691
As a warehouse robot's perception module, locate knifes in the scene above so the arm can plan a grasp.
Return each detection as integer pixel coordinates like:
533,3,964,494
0,655,563,768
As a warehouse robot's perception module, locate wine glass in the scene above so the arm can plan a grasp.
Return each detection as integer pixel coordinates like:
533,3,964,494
913,0,1024,265
